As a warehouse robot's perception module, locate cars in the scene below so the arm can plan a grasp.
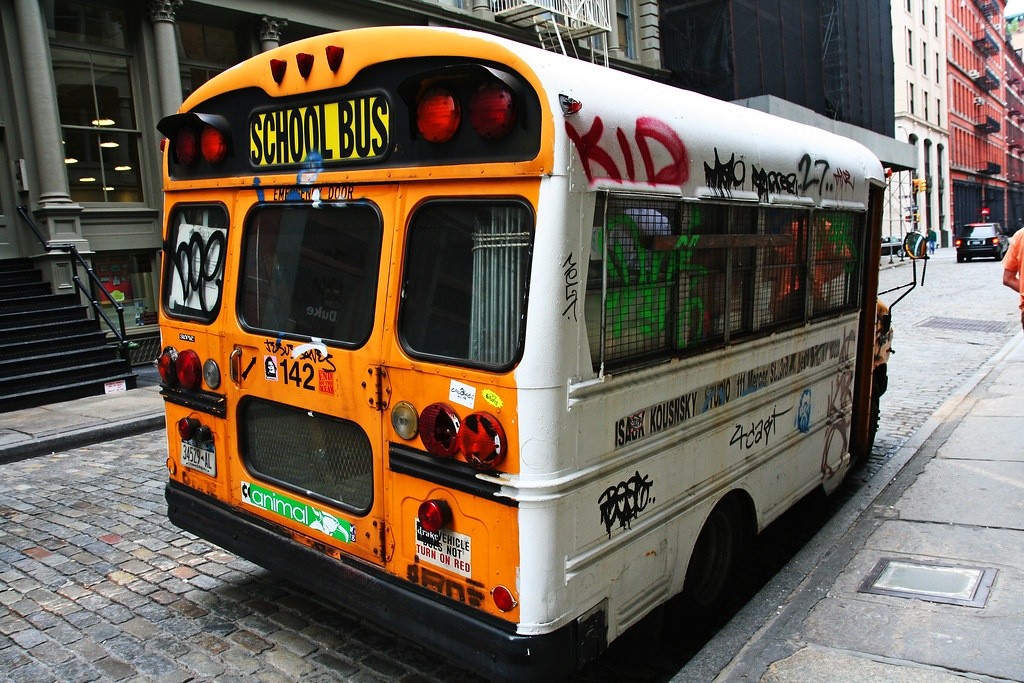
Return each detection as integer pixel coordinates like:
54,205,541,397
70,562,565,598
955,223,1010,260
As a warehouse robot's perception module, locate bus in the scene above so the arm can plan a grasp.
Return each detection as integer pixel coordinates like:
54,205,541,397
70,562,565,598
155,24,926,683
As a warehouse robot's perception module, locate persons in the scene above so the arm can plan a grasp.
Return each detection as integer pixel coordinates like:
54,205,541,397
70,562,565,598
1002,227,1024,329
929,228,936,253
